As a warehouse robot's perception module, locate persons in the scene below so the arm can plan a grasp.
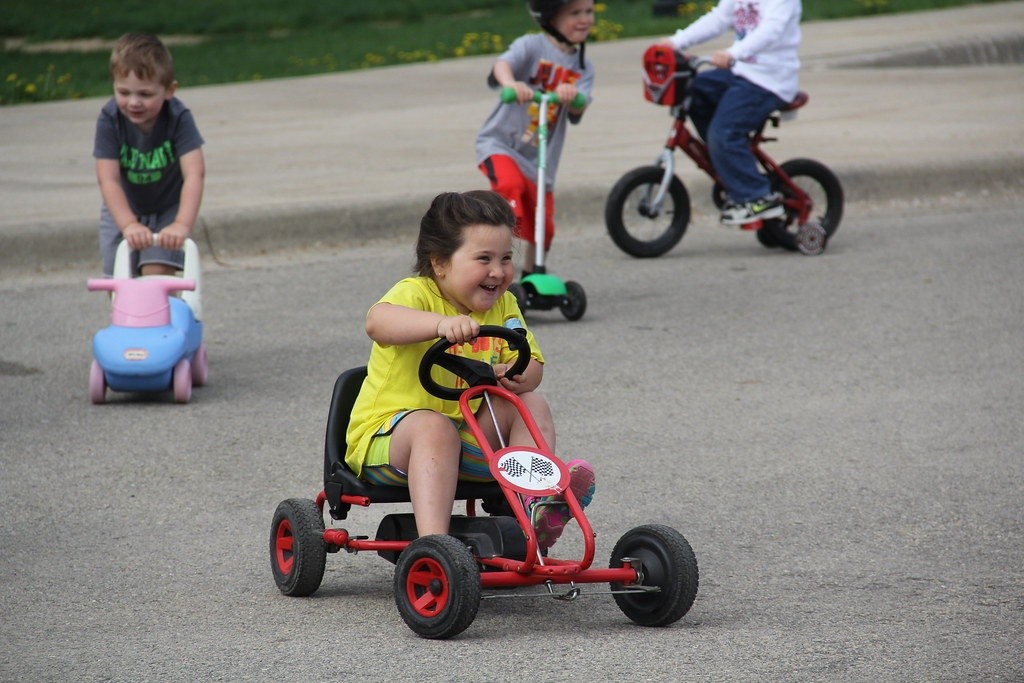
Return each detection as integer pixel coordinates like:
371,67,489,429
474,0,595,280
343,189,596,552
658,0,803,225
91,30,206,301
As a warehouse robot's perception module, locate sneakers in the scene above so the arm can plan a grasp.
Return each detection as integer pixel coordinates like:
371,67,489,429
526,459,596,552
719,191,785,226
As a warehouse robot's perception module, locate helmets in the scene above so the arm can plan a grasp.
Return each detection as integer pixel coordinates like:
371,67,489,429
527,0,572,26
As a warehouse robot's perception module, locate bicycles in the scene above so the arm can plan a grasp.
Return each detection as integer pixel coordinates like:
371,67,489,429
604,43,846,257
